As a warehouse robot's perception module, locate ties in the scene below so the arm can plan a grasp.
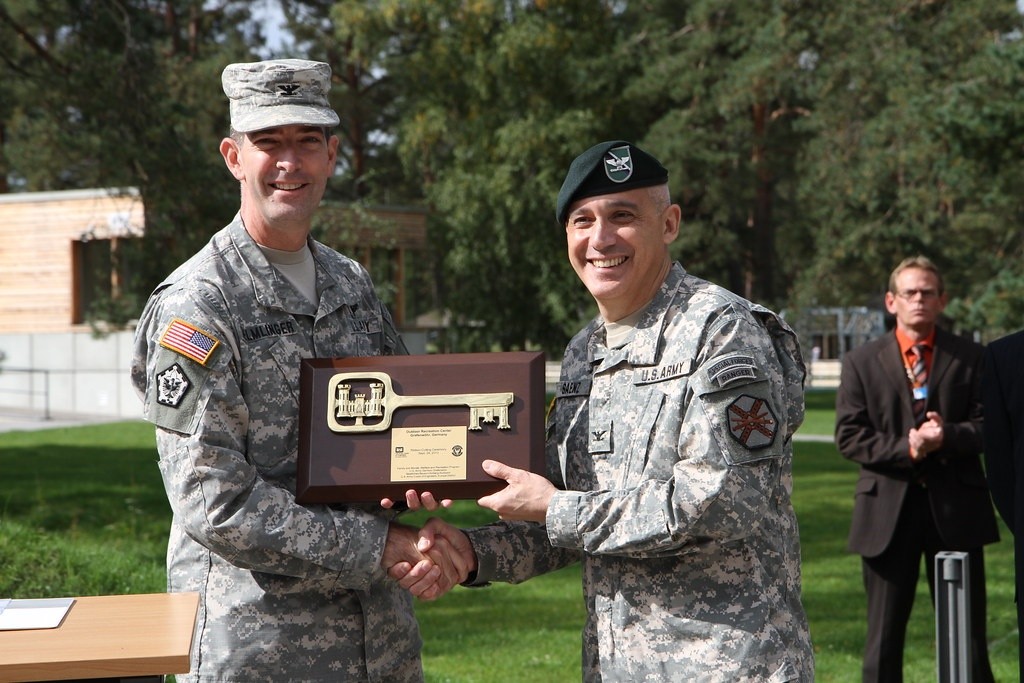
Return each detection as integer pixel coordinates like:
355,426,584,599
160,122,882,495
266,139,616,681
909,344,928,428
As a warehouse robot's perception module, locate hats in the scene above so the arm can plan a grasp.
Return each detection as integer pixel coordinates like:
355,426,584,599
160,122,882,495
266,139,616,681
556,139,668,225
222,58,342,133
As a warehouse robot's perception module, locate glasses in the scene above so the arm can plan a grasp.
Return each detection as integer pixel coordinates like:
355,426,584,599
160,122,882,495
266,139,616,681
898,289,940,300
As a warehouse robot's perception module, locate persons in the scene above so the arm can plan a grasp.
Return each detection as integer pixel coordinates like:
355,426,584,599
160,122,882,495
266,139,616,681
132,59,467,683
382,141,821,683
833,255,1024,683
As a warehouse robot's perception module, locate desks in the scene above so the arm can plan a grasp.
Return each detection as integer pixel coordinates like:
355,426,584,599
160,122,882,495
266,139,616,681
0,590,200,683
